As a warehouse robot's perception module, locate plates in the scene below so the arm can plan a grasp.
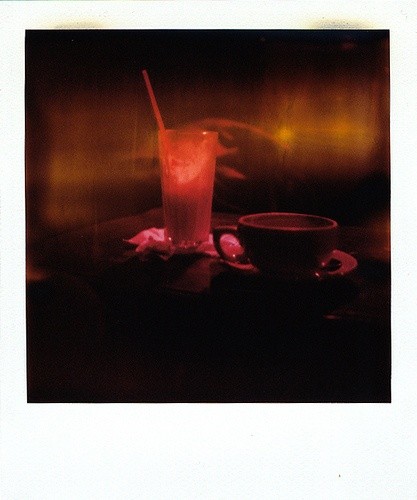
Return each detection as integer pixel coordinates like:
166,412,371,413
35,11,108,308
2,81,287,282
220,249,358,282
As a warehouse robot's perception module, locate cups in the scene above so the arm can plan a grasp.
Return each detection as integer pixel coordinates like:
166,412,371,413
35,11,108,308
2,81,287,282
213,212,338,277
158,128,219,255
